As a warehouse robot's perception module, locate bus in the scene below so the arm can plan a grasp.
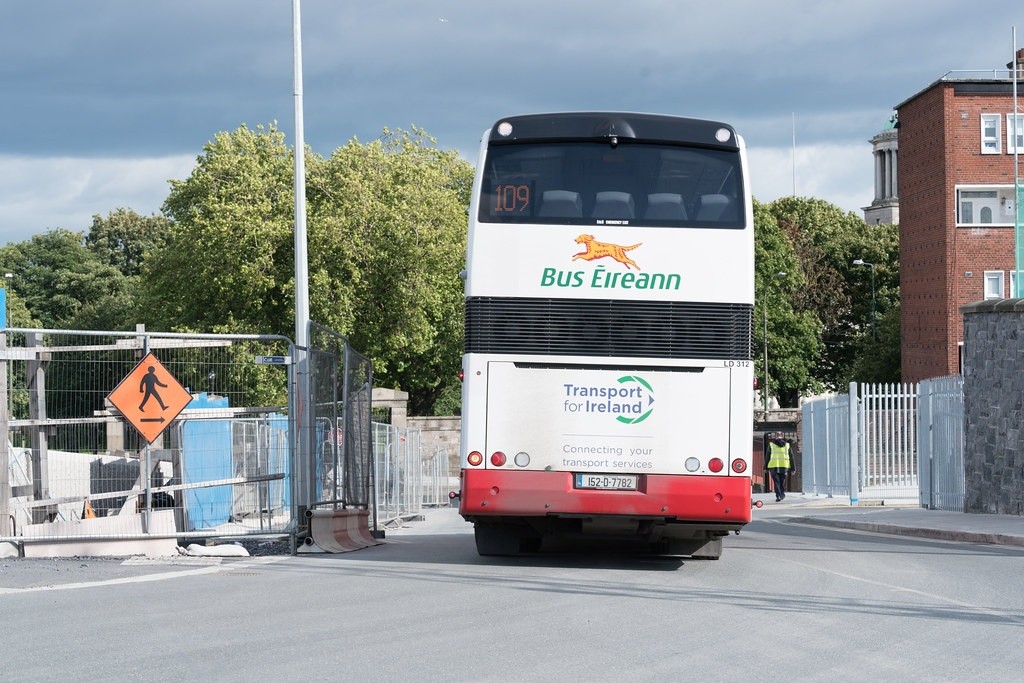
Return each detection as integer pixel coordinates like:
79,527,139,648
448,111,763,560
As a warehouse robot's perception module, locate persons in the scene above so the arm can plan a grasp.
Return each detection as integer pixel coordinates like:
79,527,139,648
763,432,796,502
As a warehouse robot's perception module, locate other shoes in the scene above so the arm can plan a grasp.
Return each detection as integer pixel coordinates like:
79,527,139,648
776,494,786,502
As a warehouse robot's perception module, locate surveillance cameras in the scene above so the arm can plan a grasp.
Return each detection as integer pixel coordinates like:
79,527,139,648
611,137,617,146
889,115,895,123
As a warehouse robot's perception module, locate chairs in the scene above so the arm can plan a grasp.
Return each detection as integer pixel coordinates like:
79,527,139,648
643,193,689,220
591,191,636,219
693,193,739,222
537,189,585,219
488,189,530,217
